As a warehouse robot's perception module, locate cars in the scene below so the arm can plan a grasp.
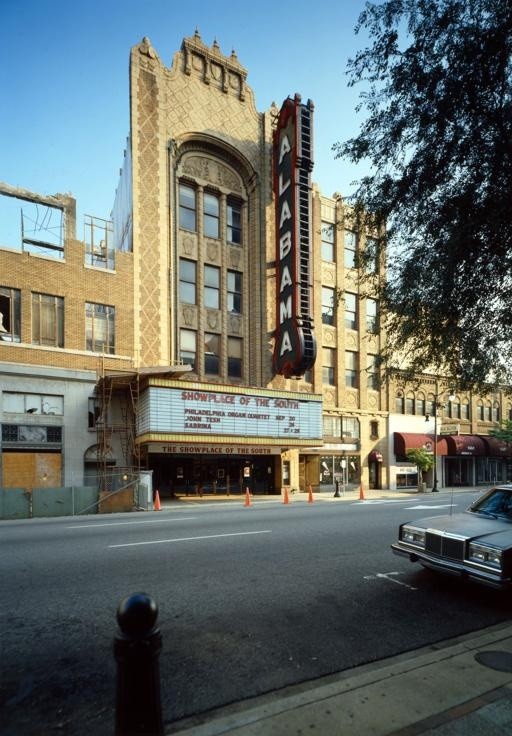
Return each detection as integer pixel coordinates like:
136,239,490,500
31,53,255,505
388,482,511,596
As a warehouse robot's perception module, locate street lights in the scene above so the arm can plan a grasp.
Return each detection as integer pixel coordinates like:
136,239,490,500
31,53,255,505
425,378,460,494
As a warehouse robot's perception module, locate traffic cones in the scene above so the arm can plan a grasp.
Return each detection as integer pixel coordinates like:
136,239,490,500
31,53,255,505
151,488,162,512
357,484,366,499
307,484,316,504
281,485,291,504
243,486,254,508
